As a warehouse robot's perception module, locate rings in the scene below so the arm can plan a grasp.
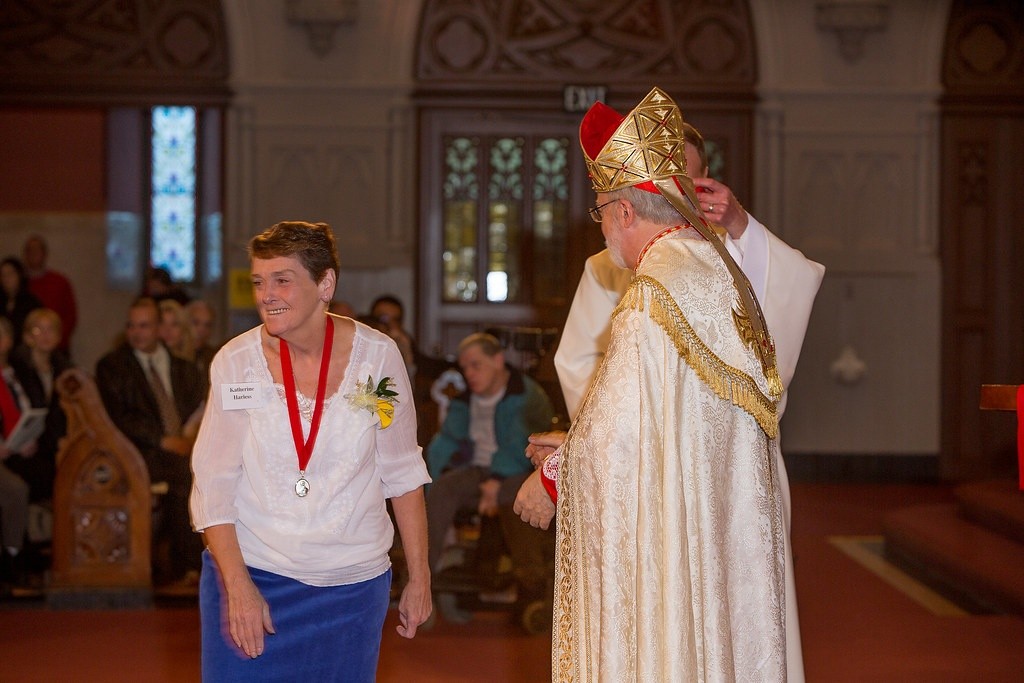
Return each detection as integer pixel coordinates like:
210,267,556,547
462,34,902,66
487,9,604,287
709,203,714,212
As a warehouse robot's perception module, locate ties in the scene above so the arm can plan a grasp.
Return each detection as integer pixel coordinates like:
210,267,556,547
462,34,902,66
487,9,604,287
147,357,181,438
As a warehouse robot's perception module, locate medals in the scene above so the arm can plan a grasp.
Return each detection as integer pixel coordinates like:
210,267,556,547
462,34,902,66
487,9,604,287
293,470,311,498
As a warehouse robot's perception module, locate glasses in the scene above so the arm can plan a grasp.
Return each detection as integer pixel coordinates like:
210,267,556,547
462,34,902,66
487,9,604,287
588,198,633,224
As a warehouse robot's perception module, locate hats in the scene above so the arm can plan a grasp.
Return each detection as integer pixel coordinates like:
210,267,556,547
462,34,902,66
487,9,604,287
579,86,776,370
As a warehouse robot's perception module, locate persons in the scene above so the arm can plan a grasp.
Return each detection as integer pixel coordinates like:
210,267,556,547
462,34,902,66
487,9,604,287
187,219,434,683
551,122,828,683
510,170,787,683
0,234,554,621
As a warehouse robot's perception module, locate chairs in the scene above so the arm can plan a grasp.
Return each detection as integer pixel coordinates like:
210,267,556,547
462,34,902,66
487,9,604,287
46,368,197,606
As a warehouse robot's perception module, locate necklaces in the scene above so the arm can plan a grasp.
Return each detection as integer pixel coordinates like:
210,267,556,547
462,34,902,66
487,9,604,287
293,364,317,425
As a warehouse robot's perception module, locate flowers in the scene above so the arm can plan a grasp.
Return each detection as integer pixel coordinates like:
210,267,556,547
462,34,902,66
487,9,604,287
343,374,401,430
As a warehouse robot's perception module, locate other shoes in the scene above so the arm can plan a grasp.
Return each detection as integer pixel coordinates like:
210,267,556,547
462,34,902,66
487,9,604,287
518,592,546,635
